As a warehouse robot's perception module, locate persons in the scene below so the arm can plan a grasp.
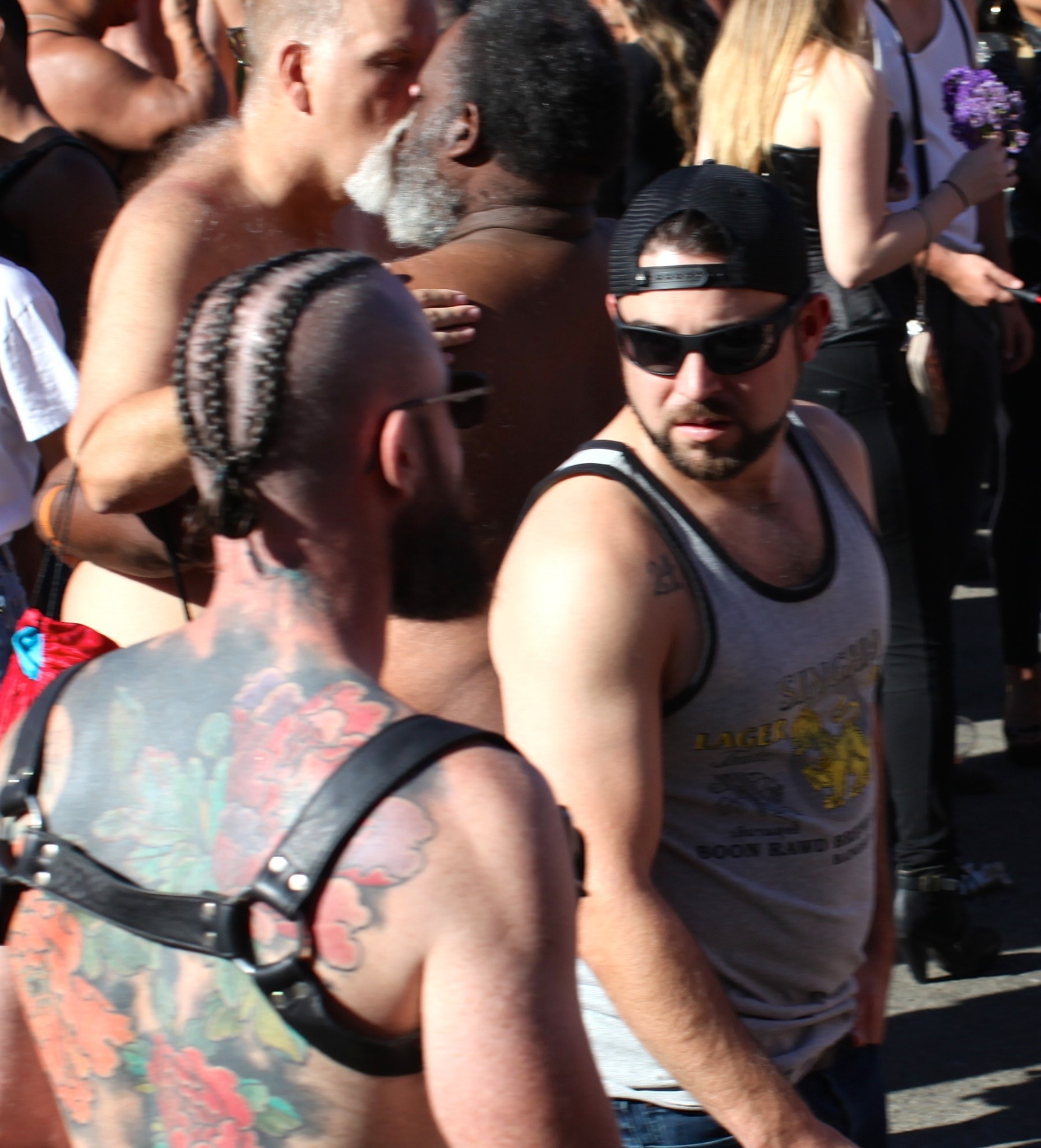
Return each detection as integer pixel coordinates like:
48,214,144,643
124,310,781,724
0,1,1041,1148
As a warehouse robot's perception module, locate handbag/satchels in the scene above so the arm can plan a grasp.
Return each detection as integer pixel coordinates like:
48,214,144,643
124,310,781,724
793,316,919,421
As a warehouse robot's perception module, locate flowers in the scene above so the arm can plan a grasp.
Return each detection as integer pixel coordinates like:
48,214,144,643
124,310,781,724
940,67,1030,153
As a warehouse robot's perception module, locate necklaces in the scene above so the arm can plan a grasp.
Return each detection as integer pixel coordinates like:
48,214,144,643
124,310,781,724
26,28,75,37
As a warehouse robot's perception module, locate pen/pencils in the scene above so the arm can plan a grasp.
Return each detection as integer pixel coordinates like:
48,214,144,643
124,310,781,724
1009,288,1041,303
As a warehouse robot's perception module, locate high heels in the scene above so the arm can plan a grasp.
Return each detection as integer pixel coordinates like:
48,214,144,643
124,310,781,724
892,887,999,982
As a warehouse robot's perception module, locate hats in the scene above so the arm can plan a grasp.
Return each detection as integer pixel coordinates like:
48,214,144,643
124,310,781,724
607,158,812,292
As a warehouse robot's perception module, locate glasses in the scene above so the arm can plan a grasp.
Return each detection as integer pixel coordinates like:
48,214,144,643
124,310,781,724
614,295,803,376
365,370,500,468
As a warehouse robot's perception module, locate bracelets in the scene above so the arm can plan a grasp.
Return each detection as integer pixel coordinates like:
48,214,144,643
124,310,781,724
940,175,971,214
910,202,930,270
